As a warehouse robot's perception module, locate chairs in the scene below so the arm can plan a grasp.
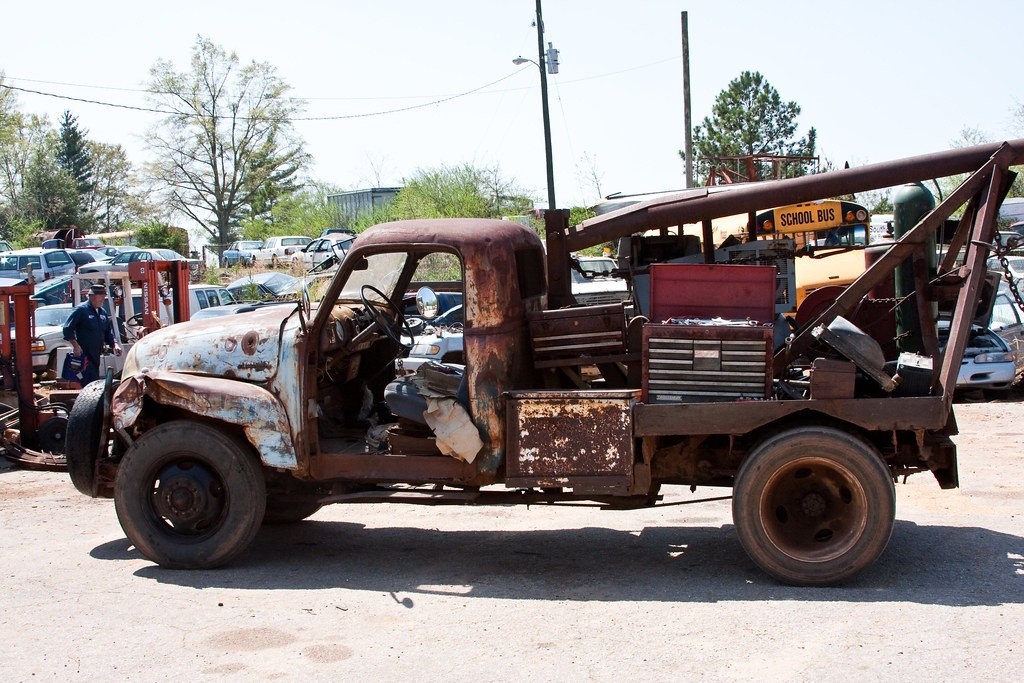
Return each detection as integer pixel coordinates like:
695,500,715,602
382,362,469,428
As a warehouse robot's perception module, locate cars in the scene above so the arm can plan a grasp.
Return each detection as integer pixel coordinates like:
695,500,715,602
221,228,356,272
571,255,634,306
0,226,307,375
937,220,1024,403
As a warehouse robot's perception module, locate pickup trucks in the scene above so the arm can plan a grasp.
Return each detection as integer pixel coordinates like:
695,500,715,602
65,139,1024,588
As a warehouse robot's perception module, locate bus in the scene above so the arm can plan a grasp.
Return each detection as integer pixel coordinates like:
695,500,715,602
642,198,872,332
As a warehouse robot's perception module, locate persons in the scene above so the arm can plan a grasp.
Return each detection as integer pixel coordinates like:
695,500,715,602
63,285,122,389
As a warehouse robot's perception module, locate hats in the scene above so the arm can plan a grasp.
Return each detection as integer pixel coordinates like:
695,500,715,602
88,284,107,295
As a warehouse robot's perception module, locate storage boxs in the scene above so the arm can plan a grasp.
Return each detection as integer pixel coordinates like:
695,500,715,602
641,264,779,404
527,302,626,360
504,388,643,478
809,357,856,399
